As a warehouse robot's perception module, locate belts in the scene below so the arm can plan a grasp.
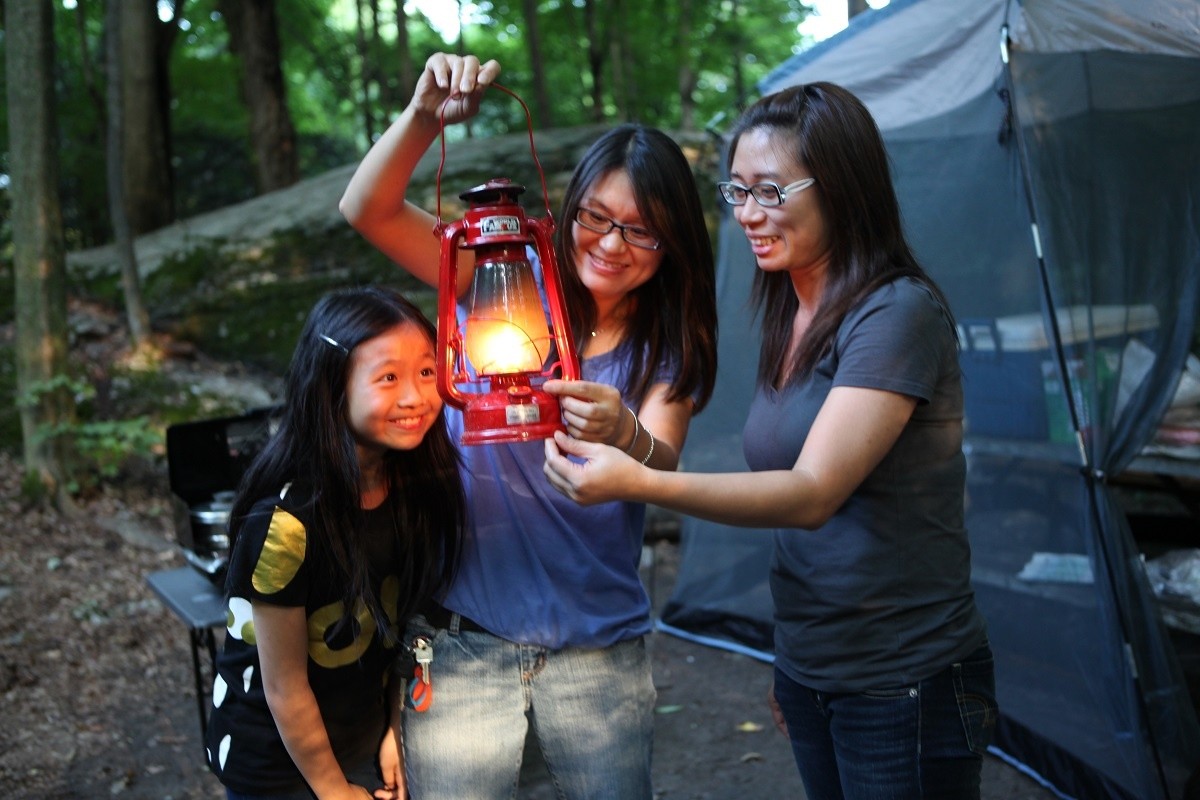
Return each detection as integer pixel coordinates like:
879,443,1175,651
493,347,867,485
415,598,485,632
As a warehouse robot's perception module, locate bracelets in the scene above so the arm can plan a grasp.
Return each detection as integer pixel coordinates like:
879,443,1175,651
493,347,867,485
626,408,640,454
642,425,655,466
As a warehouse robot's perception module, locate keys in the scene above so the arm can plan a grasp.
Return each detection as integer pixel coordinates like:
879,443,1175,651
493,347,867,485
415,639,433,685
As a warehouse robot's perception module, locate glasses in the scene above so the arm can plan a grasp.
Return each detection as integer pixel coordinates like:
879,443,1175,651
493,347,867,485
718,178,818,209
573,206,663,250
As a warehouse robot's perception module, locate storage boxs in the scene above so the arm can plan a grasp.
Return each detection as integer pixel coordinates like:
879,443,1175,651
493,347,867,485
1040,347,1120,444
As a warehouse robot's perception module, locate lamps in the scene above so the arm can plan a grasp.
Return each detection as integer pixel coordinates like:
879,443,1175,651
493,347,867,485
432,83,580,447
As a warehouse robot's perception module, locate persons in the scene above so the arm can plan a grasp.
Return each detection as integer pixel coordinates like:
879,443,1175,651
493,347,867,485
544,80,998,800
205,288,465,800
339,52,720,800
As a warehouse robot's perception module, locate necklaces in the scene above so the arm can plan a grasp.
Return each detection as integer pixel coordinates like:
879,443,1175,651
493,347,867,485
591,314,623,337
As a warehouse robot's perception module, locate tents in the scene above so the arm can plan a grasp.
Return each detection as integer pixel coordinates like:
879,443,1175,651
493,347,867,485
653,0,1200,800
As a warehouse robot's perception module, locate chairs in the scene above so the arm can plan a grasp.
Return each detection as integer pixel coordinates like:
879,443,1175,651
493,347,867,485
144,403,287,771
